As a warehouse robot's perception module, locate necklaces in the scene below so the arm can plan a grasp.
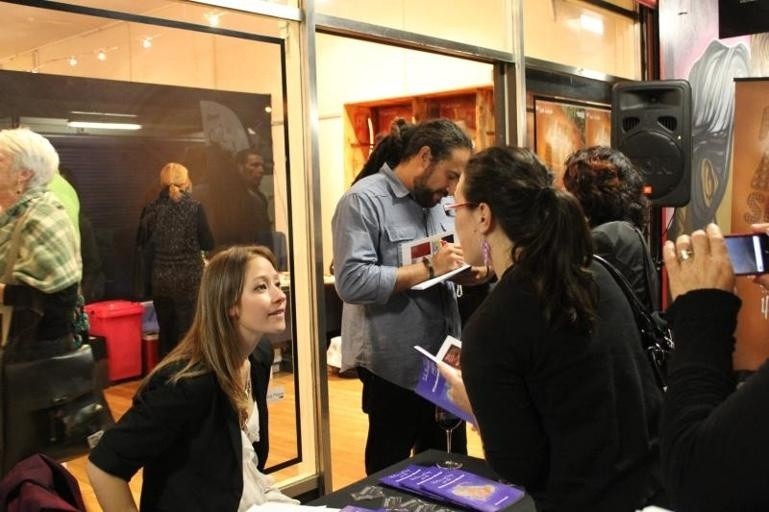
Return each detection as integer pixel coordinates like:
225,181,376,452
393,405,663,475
242,374,251,399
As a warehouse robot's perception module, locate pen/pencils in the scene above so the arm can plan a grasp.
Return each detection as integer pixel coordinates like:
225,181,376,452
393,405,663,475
440,240,447,247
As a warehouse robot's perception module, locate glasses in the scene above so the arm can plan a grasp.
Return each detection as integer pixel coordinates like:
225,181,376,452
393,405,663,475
442,203,479,216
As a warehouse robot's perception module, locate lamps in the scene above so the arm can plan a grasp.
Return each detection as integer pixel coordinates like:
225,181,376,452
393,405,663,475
68,122,143,131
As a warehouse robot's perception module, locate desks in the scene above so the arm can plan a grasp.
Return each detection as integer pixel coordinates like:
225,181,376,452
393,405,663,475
304,448,538,512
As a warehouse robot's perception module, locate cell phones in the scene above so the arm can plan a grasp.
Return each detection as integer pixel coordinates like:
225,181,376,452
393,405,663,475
717,233,769,277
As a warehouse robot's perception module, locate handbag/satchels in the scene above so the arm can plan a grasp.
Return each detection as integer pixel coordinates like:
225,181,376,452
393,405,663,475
133,246,148,299
649,306,669,333
589,252,672,400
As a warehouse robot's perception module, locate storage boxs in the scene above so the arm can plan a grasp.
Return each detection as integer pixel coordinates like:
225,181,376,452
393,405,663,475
141,330,160,374
84,300,145,381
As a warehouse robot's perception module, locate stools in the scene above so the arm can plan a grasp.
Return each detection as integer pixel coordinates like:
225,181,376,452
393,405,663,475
7,346,118,472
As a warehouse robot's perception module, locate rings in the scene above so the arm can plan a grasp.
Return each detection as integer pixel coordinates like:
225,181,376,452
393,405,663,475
679,248,695,260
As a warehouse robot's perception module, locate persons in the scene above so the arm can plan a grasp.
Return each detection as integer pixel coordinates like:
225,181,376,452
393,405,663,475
556,147,663,334
133,161,215,355
660,221,768,511
84,244,301,510
1,126,77,357
448,147,669,510
218,146,274,249
332,112,468,475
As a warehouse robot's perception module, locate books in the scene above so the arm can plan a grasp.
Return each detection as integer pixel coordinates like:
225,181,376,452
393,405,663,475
341,463,526,511
414,329,481,424
402,228,471,290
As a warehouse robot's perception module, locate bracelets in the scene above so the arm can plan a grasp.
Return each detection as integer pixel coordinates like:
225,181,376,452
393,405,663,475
423,256,434,278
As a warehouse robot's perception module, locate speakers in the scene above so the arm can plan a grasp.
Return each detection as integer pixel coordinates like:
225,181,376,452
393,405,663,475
610,80,694,206
